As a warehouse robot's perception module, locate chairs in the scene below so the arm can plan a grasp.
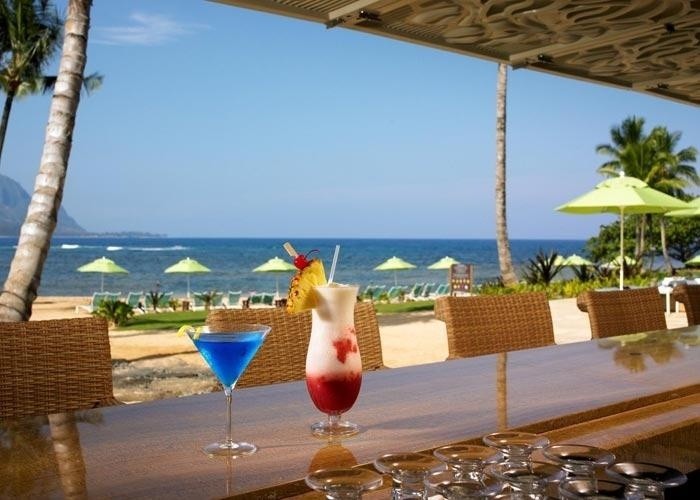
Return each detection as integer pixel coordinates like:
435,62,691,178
575,285,669,342
2,316,129,421
432,290,558,363
204,300,392,393
669,282,699,328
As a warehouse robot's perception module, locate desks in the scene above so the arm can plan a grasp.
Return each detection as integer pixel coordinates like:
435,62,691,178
2,325,700,498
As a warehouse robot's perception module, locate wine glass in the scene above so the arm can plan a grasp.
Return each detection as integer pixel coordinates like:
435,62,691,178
305,431,687,499
304,283,364,438
187,324,273,460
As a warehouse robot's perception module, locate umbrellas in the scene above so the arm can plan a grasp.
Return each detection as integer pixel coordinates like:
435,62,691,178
685,254,700,266
77,254,128,293
252,256,299,298
427,254,459,270
536,253,636,268
663,194,700,218
375,255,417,286
555,171,699,290
165,256,210,299
